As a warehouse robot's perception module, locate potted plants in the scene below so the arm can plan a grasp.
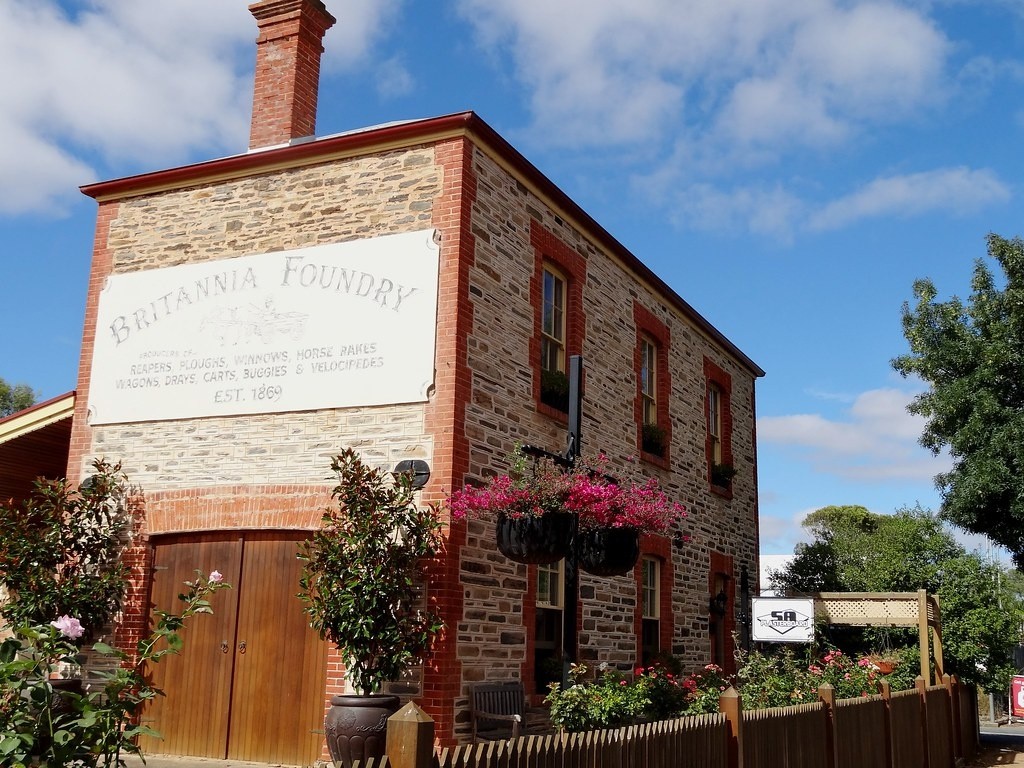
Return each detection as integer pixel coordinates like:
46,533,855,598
711,459,739,490
294,446,448,768
540,368,569,414
642,422,670,458
0,457,137,757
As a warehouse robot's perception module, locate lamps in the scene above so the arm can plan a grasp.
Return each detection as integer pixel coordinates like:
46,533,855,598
708,588,728,620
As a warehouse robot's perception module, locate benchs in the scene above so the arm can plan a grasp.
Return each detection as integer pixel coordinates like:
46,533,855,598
470,682,564,747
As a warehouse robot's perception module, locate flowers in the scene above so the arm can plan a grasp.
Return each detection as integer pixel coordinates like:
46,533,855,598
0,569,232,768
439,441,694,547
599,650,878,703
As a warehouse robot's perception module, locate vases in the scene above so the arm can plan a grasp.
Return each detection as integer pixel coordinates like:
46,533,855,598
496,511,579,563
569,527,639,576
